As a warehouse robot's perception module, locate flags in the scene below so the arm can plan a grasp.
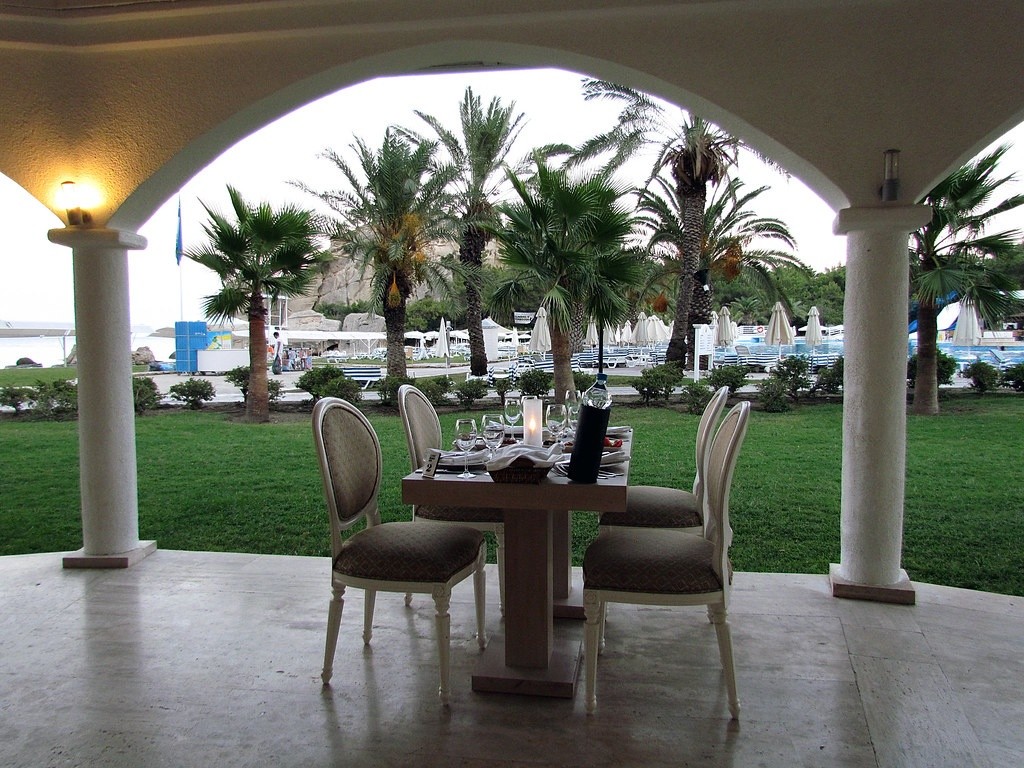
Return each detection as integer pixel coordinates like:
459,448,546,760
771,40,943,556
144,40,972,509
176,200,183,265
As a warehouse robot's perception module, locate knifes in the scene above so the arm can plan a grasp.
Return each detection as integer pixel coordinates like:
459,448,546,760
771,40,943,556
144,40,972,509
415,469,484,474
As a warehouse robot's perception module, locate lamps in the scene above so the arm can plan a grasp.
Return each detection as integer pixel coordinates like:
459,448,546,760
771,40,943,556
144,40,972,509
879,149,902,205
60,179,91,227
523,398,543,447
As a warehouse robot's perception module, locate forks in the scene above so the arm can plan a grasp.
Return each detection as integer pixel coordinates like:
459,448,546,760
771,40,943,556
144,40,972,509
551,464,624,479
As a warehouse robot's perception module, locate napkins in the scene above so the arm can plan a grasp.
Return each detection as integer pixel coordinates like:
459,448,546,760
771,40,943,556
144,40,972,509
441,425,631,464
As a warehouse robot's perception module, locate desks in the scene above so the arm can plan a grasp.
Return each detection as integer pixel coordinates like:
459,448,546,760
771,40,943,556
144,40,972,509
401,426,635,700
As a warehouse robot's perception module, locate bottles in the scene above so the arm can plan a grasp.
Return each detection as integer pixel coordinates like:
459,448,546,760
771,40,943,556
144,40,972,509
567,373,612,484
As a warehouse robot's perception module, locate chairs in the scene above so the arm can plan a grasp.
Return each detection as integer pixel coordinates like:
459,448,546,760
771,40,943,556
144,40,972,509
311,396,488,709
598,387,729,626
582,399,752,717
326,345,1015,389
398,384,506,624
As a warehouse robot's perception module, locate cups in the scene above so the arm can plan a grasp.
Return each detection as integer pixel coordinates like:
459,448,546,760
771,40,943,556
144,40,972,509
521,396,537,417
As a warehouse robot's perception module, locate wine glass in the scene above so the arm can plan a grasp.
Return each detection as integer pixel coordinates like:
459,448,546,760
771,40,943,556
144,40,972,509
504,399,520,441
455,419,478,478
546,404,567,443
568,406,580,446
565,389,581,424
481,414,504,475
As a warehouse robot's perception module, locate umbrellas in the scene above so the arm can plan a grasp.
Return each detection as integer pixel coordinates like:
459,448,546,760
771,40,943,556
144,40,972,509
201,314,249,348
954,293,982,356
530,307,552,362
804,306,823,354
403,316,531,350
585,316,599,353
603,312,674,355
435,316,450,374
763,302,793,360
711,306,739,353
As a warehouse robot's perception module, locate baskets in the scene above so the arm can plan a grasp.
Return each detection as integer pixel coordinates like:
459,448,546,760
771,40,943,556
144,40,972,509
488,458,555,484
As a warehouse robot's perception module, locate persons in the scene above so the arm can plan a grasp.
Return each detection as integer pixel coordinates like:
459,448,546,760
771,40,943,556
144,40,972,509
417,336,429,360
273,332,283,375
266,345,312,358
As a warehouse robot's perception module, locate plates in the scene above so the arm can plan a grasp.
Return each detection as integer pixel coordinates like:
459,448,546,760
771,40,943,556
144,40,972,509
606,426,630,435
602,446,620,452
422,458,487,465
452,436,519,447
556,461,622,467
